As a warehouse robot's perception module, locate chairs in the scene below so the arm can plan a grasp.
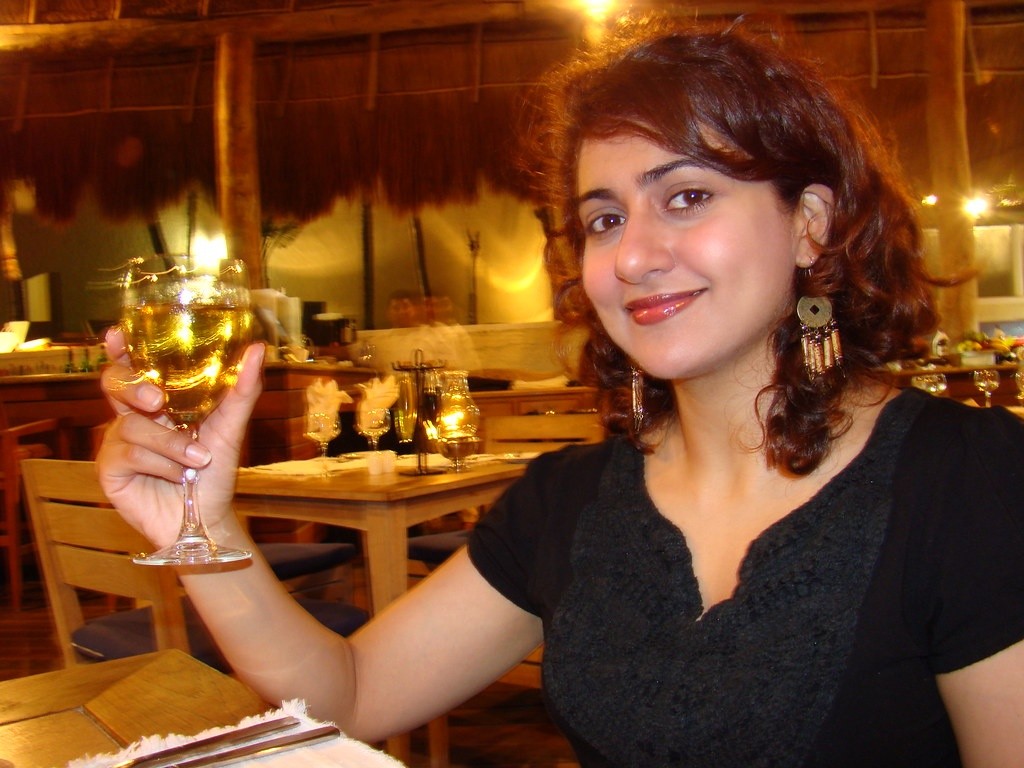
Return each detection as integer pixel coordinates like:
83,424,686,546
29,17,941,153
0,415,606,768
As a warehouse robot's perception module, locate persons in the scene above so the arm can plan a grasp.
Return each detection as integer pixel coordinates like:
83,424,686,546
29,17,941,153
95,21,1024,767
368,293,491,382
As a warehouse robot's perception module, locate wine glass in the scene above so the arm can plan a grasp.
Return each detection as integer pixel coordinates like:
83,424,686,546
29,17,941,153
304,411,341,478
121,255,253,568
441,371,478,473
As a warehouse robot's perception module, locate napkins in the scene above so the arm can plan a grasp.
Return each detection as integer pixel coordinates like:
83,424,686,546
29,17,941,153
252,457,368,475
66,697,404,768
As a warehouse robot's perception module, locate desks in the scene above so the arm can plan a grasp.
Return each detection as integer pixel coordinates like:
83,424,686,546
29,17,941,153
232,452,541,762
1,650,277,768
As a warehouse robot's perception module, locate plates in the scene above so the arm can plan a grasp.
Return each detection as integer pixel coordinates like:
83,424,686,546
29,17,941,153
493,452,543,464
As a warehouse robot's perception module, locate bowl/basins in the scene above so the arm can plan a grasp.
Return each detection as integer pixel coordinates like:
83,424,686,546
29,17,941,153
961,350,996,366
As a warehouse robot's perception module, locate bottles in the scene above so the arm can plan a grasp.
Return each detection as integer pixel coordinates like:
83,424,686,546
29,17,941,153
937,340,949,357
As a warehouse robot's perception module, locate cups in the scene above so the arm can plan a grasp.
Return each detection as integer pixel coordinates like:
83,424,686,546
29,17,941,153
974,370,1000,408
1015,368,1024,399
357,408,390,450
367,451,382,475
382,450,397,473
911,373,947,396
